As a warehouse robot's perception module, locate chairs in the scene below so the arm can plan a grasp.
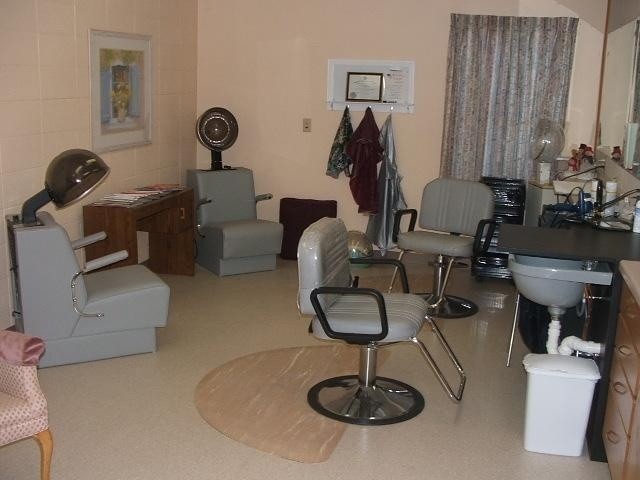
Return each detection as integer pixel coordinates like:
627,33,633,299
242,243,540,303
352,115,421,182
297,217,466,426
5,211,171,369
392,177,496,319
184,167,283,277
0,330,54,480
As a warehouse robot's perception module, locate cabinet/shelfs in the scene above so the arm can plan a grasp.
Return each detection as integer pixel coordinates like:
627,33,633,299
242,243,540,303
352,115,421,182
601,282,640,480
525,180,615,227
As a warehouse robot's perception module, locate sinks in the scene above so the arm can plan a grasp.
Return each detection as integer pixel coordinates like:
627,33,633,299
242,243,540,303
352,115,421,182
507,253,613,308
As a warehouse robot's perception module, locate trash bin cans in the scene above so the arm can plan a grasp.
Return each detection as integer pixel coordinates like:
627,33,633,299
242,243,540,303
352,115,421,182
523,354,601,457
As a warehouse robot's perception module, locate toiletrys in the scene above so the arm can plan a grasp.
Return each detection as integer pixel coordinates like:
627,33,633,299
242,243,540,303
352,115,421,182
605,180,617,216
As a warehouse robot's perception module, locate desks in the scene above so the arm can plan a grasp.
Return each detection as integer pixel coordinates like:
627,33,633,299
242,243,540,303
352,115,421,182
83,186,194,276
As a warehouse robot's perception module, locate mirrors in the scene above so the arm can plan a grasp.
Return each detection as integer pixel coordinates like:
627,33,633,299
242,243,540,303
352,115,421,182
595,14,640,180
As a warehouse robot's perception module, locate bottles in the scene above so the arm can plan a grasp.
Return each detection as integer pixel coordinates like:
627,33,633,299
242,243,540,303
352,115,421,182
604,181,618,218
632,201,640,233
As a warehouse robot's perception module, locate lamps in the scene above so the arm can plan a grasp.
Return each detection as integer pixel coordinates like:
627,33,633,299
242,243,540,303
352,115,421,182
22,148,111,228
531,120,566,184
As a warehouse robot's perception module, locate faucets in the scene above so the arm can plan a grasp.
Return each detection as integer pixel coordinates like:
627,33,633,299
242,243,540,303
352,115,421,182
580,257,594,271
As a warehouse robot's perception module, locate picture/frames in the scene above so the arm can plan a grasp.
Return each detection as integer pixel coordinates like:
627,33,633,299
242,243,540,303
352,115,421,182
345,72,383,103
87,28,153,154
